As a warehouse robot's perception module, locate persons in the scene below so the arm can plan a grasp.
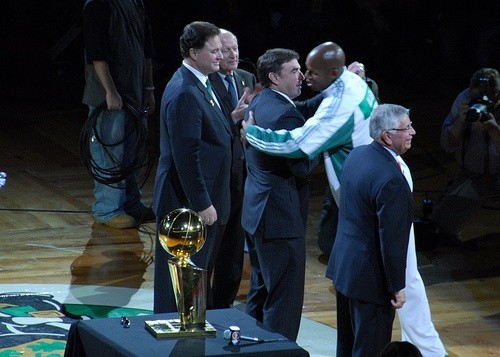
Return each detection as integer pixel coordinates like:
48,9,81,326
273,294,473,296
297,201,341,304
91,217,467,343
440,68,500,196
151,21,448,357
81,0,156,228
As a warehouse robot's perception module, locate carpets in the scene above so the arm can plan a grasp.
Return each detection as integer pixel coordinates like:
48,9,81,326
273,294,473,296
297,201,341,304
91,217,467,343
0,283,336,357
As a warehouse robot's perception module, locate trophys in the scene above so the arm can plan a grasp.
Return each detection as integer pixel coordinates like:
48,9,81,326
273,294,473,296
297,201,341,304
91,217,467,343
145,208,218,338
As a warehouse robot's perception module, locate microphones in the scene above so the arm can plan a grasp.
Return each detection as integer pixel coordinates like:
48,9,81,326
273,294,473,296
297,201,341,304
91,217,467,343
222,325,264,346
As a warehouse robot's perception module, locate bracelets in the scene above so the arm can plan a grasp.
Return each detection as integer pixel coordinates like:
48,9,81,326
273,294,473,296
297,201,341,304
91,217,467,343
144,86,155,90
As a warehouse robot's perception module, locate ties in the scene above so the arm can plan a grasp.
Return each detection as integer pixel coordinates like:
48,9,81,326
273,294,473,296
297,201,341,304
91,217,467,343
206,79,213,99
224,75,238,109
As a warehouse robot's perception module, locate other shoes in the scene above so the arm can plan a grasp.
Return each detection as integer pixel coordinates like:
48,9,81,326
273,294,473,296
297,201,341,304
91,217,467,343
94,213,136,228
129,205,156,220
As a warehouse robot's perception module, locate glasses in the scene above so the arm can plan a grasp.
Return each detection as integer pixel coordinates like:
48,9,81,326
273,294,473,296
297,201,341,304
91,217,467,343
391,123,411,132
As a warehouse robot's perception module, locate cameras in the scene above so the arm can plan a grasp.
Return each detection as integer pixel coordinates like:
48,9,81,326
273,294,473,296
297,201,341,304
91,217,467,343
465,77,493,124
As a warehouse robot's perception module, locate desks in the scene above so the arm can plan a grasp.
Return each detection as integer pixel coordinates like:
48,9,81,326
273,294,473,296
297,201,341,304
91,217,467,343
63,307,310,357
